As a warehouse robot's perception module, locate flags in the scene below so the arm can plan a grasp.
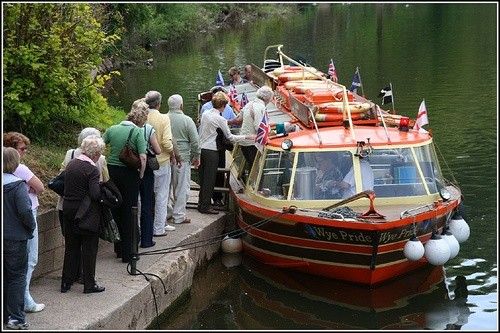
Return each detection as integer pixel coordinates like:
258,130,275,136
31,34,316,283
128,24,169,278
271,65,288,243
378,83,393,105
230,84,238,102
413,98,429,129
255,110,269,144
216,69,225,86
350,67,361,91
327,58,339,83
263,59,281,73
241,92,247,106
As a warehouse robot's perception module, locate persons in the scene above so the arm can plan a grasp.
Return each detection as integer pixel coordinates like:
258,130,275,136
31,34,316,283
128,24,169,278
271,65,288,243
100,92,175,248
3,146,36,330
3,132,45,312
200,86,242,206
144,91,177,236
55,127,110,284
165,94,202,223
100,110,148,262
61,135,106,293
236,85,275,165
335,154,375,199
314,152,342,199
129,102,162,248
243,64,252,81
197,95,257,213
227,66,247,86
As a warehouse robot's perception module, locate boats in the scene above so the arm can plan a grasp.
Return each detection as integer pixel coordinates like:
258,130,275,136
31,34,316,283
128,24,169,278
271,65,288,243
226,253,451,330
197,44,461,287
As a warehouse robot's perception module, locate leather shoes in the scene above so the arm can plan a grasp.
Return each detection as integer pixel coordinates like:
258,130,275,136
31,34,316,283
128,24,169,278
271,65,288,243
83,285,105,293
61,284,70,292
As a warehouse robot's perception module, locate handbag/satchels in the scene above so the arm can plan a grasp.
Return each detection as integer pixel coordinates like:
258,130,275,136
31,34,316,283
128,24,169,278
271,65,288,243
47,171,64,197
119,128,139,168
144,124,159,169
100,218,120,242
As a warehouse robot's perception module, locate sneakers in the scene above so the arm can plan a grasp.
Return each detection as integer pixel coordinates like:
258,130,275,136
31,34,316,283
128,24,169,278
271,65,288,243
6,316,29,329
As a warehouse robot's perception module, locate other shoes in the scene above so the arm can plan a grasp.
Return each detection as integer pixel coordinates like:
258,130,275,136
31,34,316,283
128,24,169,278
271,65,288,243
167,217,172,220
31,304,45,312
154,233,168,236
183,218,190,223
117,253,121,257
207,208,219,214
143,241,156,247
122,257,139,262
166,225,176,230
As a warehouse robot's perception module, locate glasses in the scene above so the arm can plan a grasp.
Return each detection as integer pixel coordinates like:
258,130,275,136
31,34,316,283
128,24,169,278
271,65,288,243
15,147,27,151
230,73,236,76
